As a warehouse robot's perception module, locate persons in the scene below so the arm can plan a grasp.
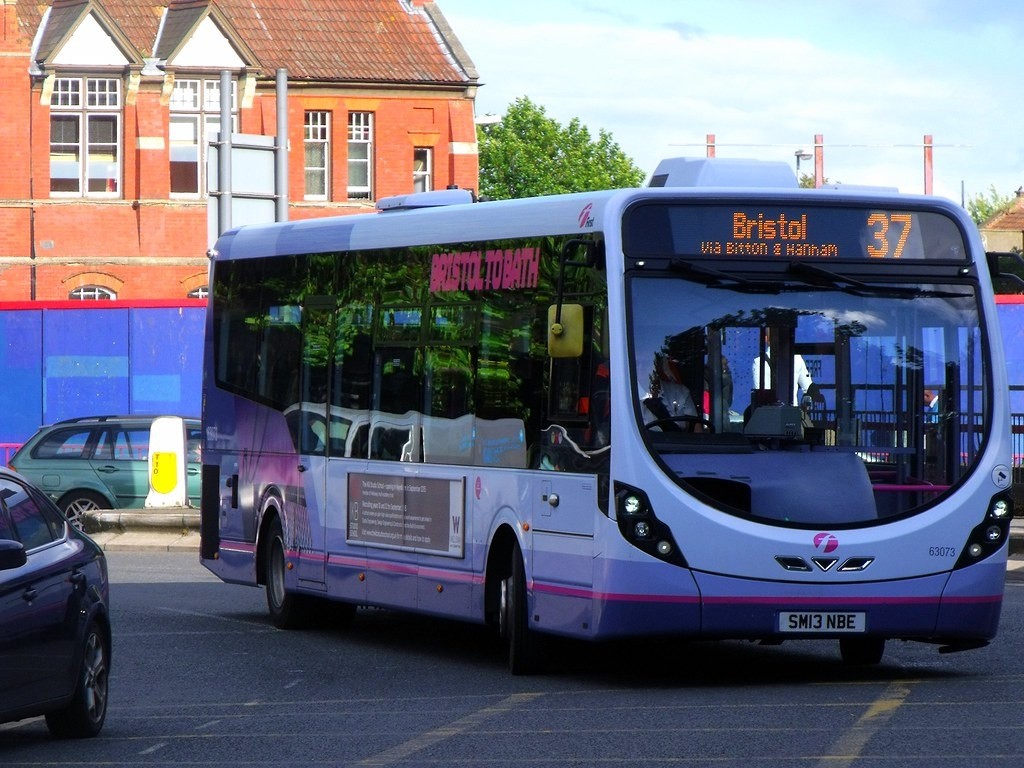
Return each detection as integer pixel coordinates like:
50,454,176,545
636,349,706,433
752,324,824,439
925,389,940,425
720,353,734,434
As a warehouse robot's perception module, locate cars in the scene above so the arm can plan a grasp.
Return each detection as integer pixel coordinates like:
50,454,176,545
0,465,111,745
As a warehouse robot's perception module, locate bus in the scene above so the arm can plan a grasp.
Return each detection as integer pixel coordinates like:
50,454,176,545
198,158,1024,685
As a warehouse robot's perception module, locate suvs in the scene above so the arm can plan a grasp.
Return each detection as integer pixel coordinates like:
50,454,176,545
9,413,200,532
